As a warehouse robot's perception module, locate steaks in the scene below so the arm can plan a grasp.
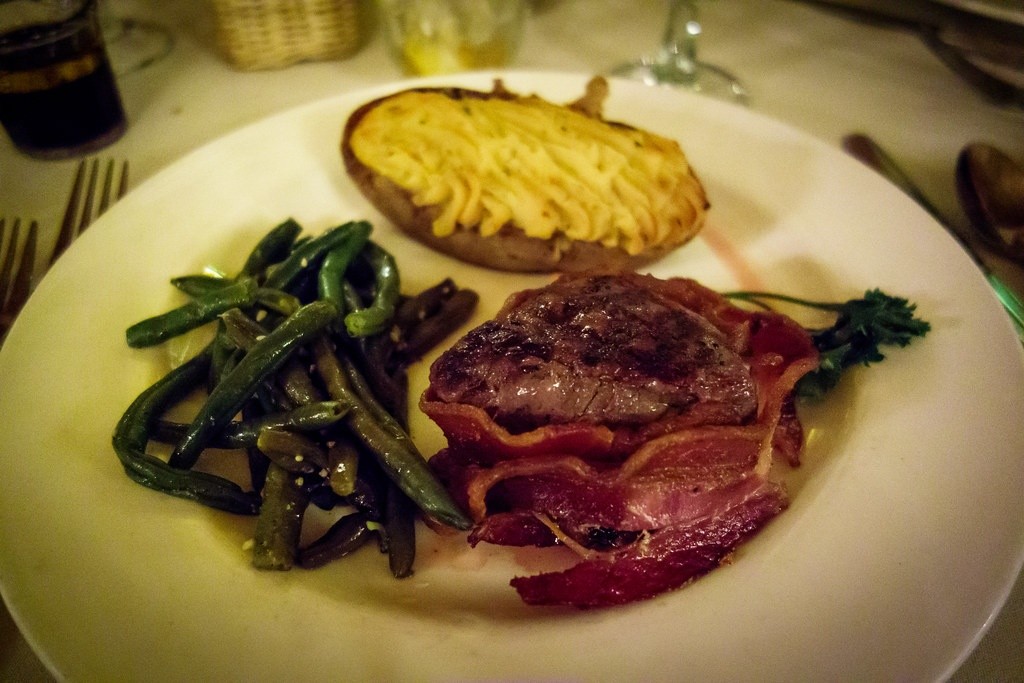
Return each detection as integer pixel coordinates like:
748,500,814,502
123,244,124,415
340,88,707,276
428,274,759,426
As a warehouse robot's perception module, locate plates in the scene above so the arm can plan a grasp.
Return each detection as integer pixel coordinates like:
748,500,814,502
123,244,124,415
0,65,1022,682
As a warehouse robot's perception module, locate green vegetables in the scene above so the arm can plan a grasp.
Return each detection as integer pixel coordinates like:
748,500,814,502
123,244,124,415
110,218,476,578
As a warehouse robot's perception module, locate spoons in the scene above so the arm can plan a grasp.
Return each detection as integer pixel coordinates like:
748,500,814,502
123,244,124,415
952,140,1024,270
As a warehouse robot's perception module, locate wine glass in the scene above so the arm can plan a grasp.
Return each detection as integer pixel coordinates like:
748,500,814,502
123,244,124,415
603,4,754,117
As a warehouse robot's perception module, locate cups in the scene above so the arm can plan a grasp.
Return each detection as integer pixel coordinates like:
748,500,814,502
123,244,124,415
376,0,531,82
0,1,129,163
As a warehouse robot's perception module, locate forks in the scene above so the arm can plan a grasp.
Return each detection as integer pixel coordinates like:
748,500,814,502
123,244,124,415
44,153,127,267
1,216,36,346
